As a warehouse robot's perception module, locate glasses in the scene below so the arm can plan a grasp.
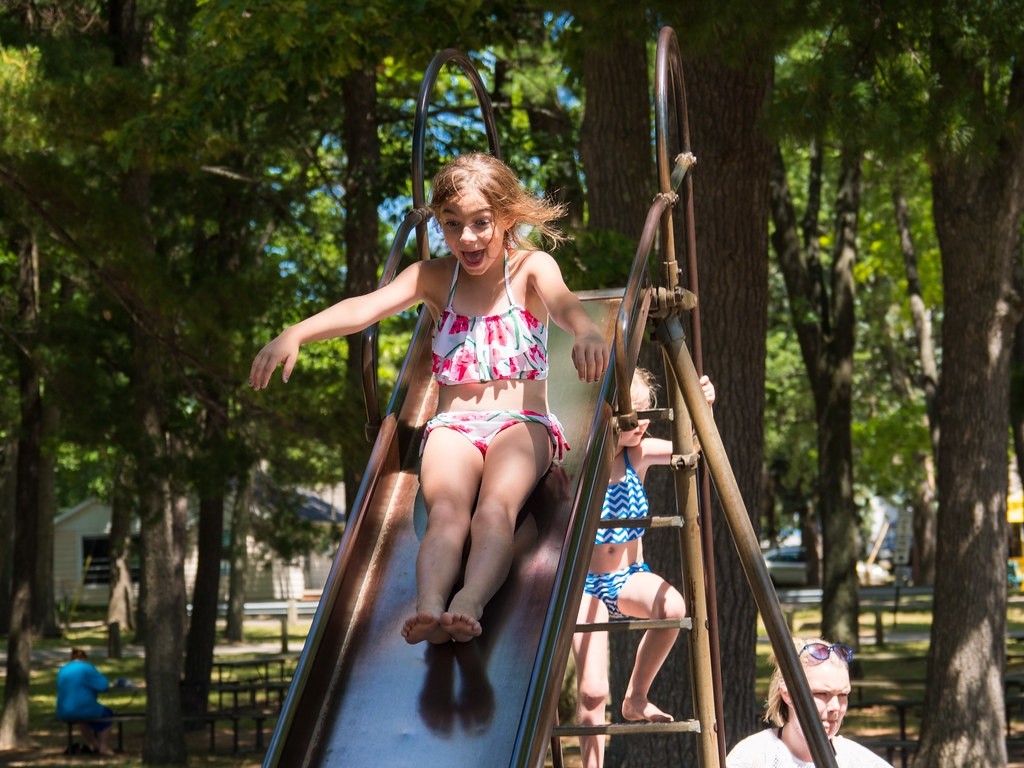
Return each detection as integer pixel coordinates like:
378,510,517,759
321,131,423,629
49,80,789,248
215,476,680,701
797,643,853,662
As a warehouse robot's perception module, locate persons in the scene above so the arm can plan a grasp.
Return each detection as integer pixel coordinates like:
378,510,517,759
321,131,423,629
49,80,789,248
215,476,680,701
55,647,115,756
726,638,895,768
247,154,610,644
571,366,716,768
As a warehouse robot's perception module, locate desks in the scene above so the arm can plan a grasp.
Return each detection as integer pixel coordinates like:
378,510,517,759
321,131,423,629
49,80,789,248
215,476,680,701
850,651,1009,706
94,680,146,711
211,652,285,711
880,691,1024,768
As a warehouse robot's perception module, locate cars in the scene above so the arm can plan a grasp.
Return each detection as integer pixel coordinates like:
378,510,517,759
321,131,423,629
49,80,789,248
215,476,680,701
760,546,812,589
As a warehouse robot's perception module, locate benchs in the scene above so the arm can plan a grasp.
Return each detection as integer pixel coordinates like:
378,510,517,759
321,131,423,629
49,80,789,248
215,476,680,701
851,632,1024,768
179,672,295,755
44,716,145,752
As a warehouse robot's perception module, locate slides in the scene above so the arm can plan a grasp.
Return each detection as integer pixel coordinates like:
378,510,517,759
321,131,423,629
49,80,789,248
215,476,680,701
258,193,672,767
